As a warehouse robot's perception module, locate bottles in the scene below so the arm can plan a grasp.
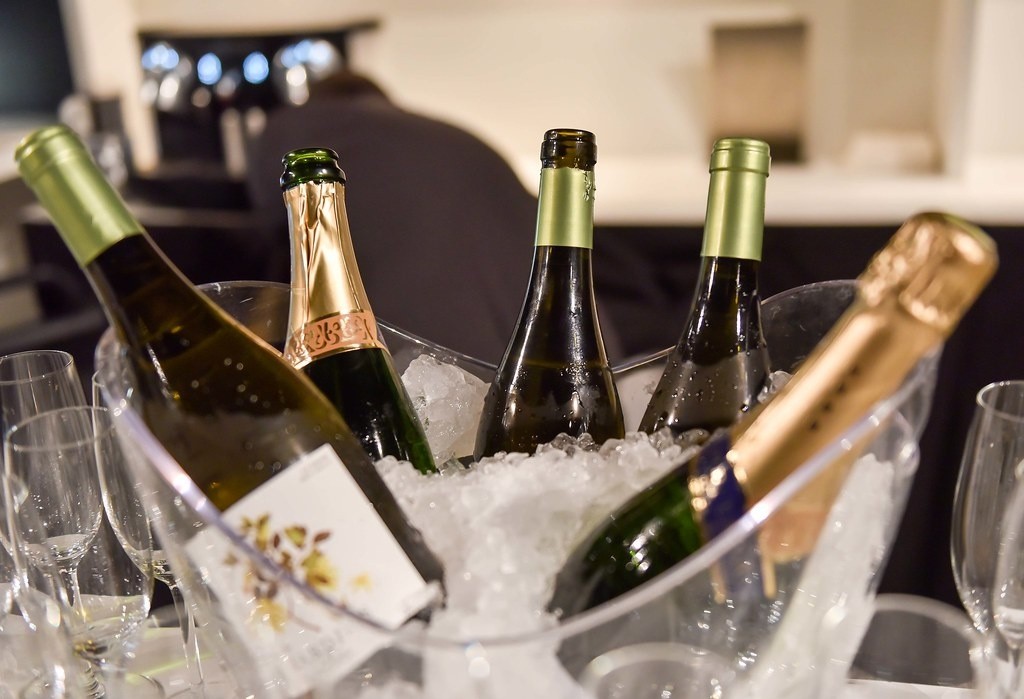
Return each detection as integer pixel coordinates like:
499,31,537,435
269,149,438,511
12,124,452,693
474,126,624,465
538,209,1001,699
638,136,779,451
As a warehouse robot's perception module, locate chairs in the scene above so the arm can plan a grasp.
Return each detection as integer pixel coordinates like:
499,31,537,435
253,105,620,388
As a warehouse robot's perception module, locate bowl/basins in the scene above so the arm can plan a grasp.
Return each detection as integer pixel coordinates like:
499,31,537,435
91,278,941,699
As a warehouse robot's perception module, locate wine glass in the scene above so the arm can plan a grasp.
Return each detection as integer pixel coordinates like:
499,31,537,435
0,349,104,634
948,378,1024,699
4,406,168,699
